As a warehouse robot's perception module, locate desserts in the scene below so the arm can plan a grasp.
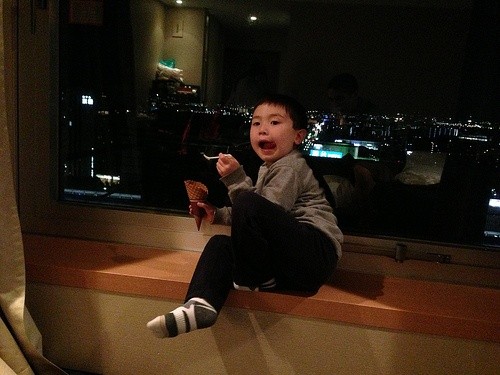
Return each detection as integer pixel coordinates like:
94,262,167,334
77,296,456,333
183,180,209,231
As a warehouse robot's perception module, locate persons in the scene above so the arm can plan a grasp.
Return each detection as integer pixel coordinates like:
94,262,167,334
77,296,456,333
145,97,345,340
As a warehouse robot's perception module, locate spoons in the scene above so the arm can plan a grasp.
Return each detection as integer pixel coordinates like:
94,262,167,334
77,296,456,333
202,152,232,159
